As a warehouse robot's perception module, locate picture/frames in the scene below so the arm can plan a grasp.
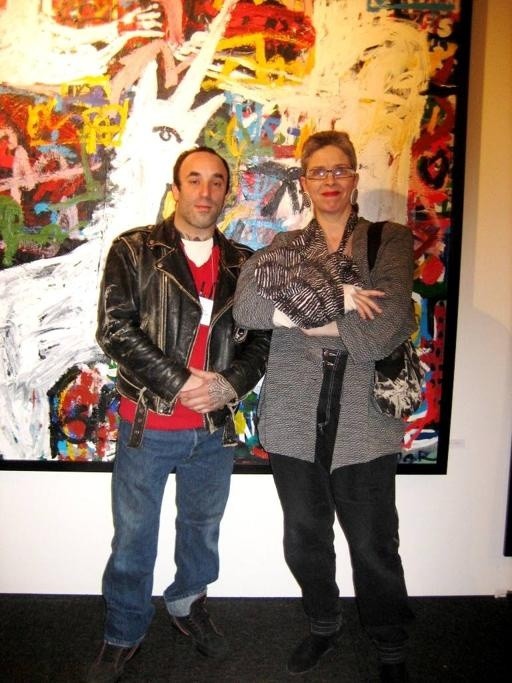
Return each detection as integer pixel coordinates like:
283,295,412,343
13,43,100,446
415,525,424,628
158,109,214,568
0,0,474,476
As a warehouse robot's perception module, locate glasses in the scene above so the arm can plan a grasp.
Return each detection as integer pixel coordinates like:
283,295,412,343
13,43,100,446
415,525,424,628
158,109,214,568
306,166,358,179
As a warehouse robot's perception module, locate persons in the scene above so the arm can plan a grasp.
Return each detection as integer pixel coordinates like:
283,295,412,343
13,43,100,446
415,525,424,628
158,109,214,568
232,129,414,683
85,147,272,683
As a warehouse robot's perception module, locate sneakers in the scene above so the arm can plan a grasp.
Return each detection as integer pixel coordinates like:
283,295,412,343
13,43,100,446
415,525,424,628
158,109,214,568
285,621,345,676
376,642,409,683
169,593,228,659
87,641,141,683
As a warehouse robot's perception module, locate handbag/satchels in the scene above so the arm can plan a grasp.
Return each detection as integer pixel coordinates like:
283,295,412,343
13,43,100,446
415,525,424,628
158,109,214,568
365,220,423,418
